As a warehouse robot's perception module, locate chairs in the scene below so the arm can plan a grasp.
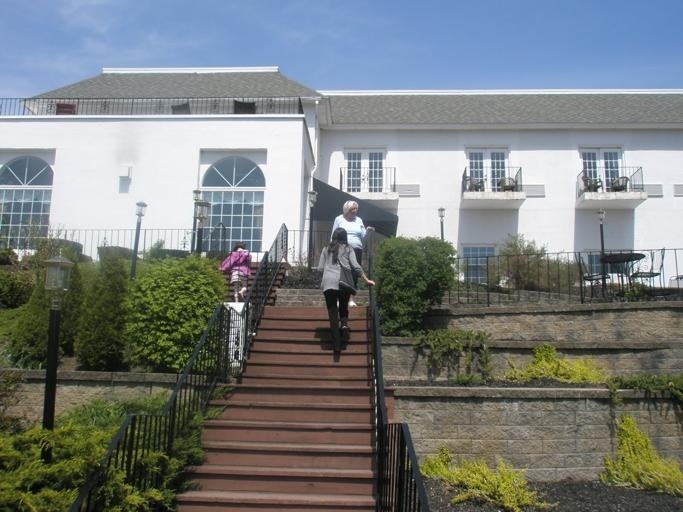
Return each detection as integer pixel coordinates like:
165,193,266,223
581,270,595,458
499,177,516,192
582,175,630,192
468,177,481,191
572,247,667,304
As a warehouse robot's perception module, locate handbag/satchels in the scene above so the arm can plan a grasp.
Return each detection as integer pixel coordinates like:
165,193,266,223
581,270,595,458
338,267,357,296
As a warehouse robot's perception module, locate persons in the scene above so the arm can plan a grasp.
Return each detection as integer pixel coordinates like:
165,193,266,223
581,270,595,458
329,201,376,307
317,227,376,353
218,242,252,303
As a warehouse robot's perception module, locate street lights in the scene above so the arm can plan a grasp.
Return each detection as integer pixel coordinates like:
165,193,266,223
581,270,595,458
40,246,76,465
597,207,607,299
130,200,148,279
191,189,212,259
437,207,446,240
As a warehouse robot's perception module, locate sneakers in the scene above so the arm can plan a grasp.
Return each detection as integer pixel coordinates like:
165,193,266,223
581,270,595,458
340,325,349,339
348,300,358,308
333,343,341,352
